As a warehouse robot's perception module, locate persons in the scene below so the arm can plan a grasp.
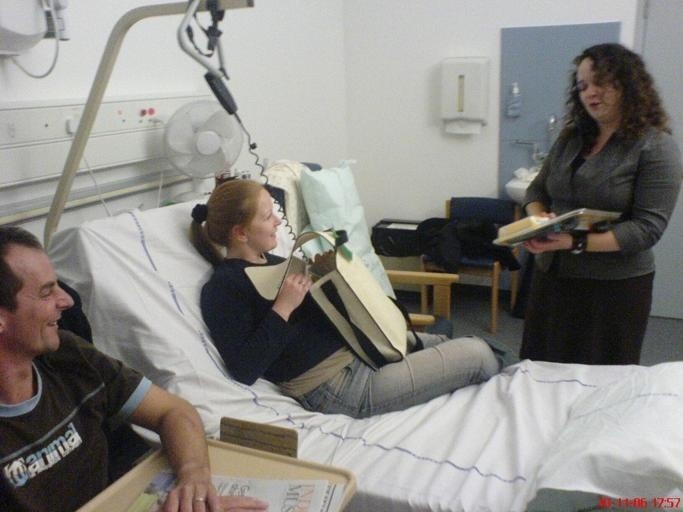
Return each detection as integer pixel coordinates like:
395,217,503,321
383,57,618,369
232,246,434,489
0,226,271,512
519,43,683,365
191,179,506,419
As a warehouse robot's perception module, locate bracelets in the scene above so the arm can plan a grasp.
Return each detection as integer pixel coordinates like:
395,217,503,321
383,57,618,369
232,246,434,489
570,230,586,256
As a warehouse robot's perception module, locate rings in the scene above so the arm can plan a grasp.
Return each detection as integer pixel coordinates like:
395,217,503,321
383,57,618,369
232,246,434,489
194,497,207,502
298,281,305,286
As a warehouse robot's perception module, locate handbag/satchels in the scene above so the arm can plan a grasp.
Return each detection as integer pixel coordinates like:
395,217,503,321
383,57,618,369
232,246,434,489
241,226,424,373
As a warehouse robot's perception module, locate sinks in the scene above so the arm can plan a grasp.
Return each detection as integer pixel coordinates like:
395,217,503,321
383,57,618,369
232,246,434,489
504,165,542,206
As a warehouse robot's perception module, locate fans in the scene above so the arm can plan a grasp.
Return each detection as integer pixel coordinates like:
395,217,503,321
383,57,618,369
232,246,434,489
161,99,244,201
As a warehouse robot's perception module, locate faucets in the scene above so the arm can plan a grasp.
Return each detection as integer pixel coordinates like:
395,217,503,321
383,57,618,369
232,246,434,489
546,114,560,140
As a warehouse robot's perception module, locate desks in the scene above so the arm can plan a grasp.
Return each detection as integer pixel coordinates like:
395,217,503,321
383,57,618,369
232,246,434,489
72,434,358,510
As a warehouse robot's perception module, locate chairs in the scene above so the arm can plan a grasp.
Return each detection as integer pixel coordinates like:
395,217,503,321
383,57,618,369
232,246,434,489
261,159,459,336
417,195,523,332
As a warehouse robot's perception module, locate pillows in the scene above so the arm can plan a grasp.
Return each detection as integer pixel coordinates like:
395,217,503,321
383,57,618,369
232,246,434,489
298,163,396,300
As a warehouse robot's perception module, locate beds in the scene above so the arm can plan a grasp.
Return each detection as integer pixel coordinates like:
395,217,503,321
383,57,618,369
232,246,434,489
46,184,682,511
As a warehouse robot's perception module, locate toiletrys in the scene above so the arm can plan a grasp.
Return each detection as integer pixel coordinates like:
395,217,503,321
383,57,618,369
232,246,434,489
504,81,524,118
